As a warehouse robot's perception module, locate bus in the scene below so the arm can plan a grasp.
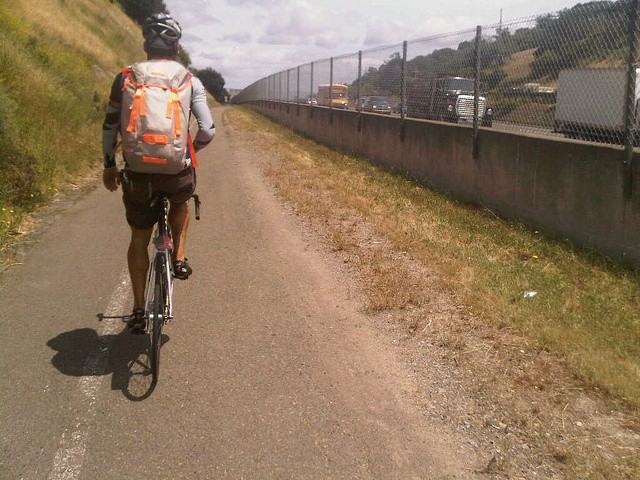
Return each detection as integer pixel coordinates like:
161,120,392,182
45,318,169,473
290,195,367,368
318,84,349,108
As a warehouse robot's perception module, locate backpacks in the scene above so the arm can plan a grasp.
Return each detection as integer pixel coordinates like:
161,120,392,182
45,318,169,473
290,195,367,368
109,62,199,175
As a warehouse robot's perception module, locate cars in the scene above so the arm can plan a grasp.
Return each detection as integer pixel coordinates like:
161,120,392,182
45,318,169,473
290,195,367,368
307,98,317,104
393,102,407,113
363,97,390,114
356,98,369,111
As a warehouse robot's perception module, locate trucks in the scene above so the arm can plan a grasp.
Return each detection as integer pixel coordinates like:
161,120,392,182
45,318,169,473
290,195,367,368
556,67,640,147
407,77,493,127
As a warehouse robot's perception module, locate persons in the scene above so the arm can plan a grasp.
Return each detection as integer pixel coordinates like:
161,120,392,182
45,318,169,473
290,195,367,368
101,12,217,331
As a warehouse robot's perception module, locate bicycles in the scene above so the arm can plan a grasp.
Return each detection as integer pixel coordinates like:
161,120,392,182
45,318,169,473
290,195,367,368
132,187,201,379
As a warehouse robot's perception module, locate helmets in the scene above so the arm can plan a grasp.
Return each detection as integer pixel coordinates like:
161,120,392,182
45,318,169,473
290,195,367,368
142,12,181,50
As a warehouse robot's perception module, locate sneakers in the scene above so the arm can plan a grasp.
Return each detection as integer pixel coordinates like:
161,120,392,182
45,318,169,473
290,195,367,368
127,309,146,329
172,257,192,277
